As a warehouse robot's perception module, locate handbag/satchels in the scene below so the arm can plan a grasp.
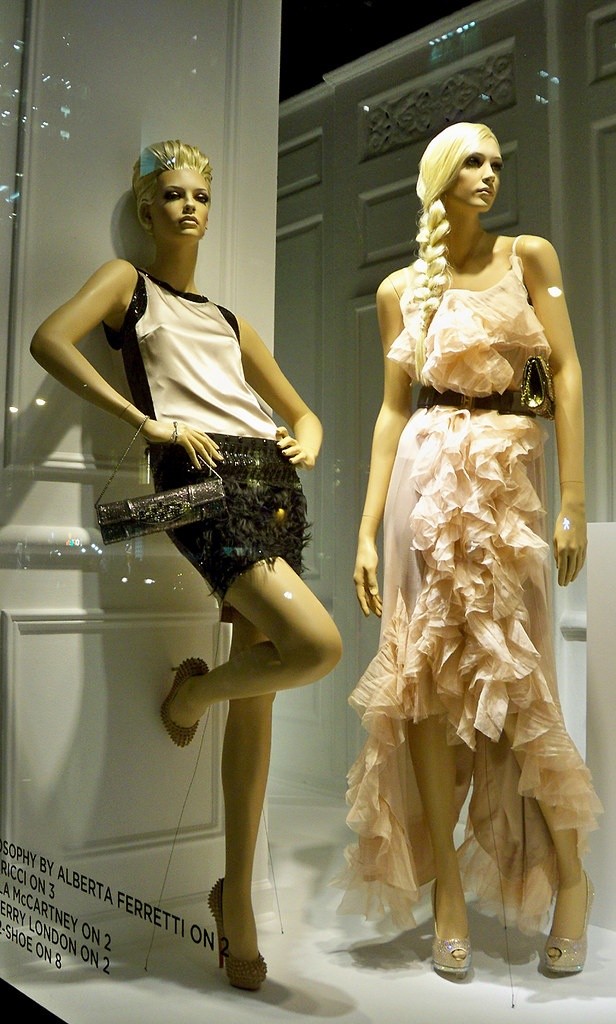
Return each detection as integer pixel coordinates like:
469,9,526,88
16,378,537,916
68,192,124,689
94,416,228,545
520,354,557,422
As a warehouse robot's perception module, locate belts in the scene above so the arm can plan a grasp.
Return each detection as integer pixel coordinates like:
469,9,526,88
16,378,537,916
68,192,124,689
416,385,538,417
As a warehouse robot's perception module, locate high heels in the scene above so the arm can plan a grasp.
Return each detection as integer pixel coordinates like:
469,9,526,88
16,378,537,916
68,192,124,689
159,657,208,747
208,877,268,989
430,877,474,974
543,870,594,971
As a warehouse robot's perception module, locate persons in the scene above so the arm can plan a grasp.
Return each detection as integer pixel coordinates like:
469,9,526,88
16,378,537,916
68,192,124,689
344,122,604,976
29,138,343,991
